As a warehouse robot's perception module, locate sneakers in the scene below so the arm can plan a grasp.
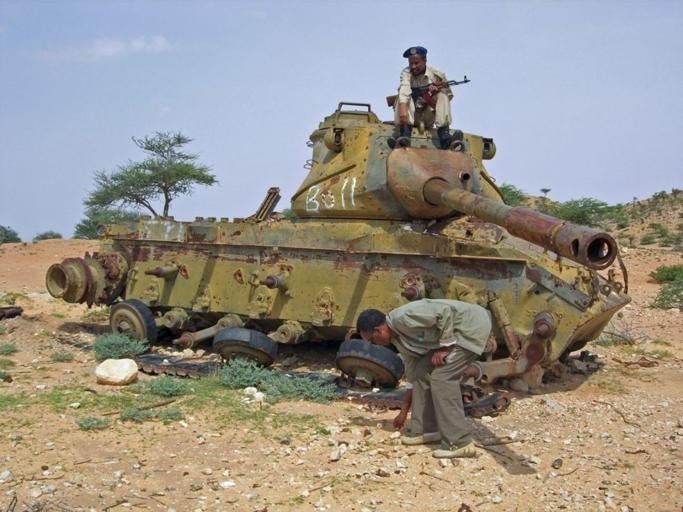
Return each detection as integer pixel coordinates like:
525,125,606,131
399,430,441,446
431,443,475,458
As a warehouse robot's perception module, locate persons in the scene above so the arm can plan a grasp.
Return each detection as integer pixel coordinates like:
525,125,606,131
385,46,464,149
356,297,492,459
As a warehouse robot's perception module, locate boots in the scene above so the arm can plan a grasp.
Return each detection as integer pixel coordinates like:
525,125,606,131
437,126,462,149
387,124,410,148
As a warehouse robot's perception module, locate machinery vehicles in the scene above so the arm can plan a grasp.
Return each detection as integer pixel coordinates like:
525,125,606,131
45,103,632,408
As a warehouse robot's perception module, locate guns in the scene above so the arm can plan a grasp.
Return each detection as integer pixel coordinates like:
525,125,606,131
386,75,471,107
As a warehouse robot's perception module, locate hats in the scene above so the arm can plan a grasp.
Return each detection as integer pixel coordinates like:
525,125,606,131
402,46,427,57
